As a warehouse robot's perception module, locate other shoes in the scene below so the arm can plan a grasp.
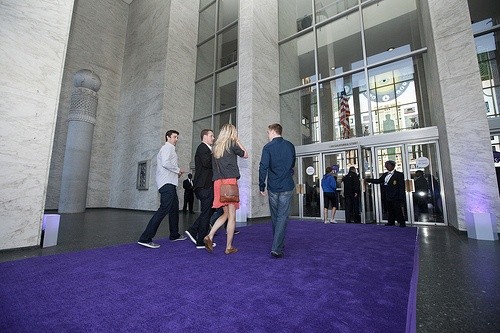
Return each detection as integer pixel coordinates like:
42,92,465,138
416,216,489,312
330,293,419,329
400,222,406,227
208,223,217,236
385,222,395,226
224,230,240,235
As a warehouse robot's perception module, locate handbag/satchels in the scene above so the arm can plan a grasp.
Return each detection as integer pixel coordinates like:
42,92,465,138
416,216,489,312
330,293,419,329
220,184,239,203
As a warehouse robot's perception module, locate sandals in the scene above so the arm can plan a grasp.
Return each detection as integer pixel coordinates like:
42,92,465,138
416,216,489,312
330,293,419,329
203,235,214,253
224,246,238,255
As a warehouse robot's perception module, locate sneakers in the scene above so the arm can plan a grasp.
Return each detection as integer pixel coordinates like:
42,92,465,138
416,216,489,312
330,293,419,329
137,238,160,248
185,228,197,245
330,220,338,223
270,248,283,257
324,220,330,224
196,243,216,249
169,232,187,242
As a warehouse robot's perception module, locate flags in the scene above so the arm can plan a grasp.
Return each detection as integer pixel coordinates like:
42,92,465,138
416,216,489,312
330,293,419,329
339,89,350,156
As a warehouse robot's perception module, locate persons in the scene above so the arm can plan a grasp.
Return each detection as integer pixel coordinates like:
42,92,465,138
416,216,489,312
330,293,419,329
365,161,407,227
183,173,196,215
259,123,297,257
138,130,188,249
321,167,337,224
364,126,372,151
413,170,429,220
409,117,421,161
203,123,249,254
428,175,442,216
185,128,217,249
304,179,319,217
340,176,344,210
344,167,361,223
